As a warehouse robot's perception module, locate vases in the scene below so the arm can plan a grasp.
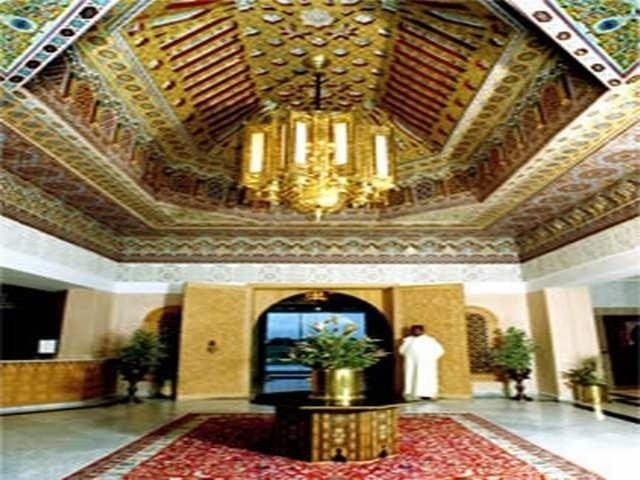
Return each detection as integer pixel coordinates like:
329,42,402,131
307,368,368,406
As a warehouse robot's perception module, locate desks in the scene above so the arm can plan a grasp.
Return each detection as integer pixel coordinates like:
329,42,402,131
249,390,410,463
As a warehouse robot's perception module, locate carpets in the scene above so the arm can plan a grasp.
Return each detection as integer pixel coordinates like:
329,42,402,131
61,409,607,479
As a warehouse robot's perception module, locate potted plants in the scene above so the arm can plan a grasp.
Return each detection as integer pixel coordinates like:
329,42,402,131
112,327,171,408
486,324,544,403
570,357,610,408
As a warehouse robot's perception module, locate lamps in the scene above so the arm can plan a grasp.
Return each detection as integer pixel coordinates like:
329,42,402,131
236,53,402,224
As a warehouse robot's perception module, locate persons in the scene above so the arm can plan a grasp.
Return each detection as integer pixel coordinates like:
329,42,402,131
397,323,445,402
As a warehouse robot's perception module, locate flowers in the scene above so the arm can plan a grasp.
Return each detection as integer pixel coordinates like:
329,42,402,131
282,316,393,371
